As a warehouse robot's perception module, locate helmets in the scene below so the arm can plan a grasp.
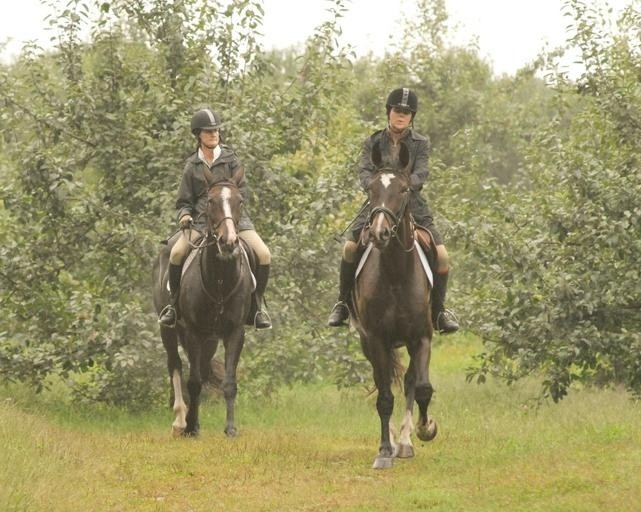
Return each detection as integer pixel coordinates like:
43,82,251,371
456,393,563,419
386,88,417,122
191,110,222,132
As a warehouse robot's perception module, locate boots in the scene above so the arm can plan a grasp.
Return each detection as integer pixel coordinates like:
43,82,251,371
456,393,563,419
247,264,270,328
161,263,182,322
431,270,459,329
328,256,357,326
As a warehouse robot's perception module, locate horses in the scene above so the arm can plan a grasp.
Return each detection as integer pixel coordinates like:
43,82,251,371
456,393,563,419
150,161,258,439
349,137,438,471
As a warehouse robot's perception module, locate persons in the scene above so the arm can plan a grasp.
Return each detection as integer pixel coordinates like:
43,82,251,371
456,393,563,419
327,87,460,333
157,107,272,331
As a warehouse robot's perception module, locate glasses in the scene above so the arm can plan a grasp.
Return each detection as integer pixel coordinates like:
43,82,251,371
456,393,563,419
394,107,410,114
